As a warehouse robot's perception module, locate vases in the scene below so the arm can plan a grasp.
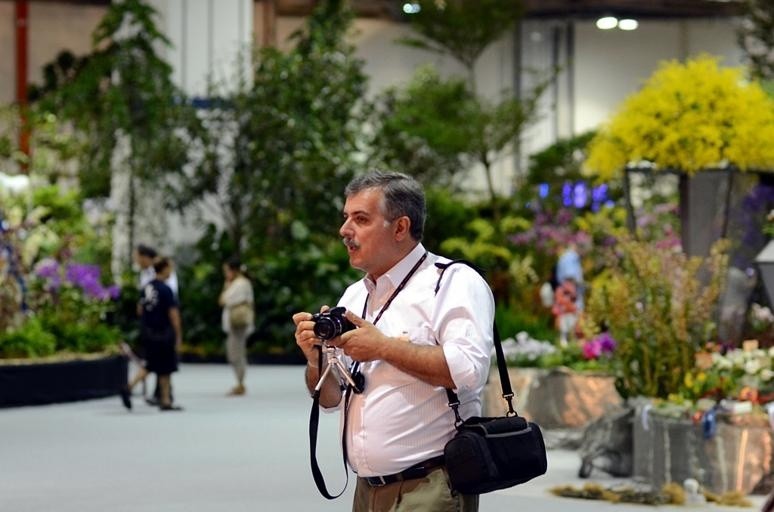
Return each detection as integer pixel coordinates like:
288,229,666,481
524,366,773,501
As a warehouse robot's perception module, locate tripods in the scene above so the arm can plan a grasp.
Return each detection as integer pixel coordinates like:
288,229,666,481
310,343,363,399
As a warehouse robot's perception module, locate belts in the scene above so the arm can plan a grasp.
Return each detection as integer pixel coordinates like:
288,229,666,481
365,454,443,487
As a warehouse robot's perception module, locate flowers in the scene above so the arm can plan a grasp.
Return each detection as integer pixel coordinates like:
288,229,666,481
492,333,774,410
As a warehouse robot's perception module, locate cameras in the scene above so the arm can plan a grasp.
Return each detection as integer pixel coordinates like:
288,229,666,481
309,306,357,342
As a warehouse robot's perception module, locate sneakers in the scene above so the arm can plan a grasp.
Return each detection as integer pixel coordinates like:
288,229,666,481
118,385,179,411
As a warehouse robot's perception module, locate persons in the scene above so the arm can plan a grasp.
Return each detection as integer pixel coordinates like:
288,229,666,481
292,169,496,511
217,258,255,395
119,243,182,410
554,242,586,346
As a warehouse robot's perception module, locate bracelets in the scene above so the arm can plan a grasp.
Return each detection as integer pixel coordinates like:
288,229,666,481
308,356,327,369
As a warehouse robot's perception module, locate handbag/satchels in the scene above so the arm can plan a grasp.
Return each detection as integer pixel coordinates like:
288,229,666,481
443,413,546,496
230,302,252,330
